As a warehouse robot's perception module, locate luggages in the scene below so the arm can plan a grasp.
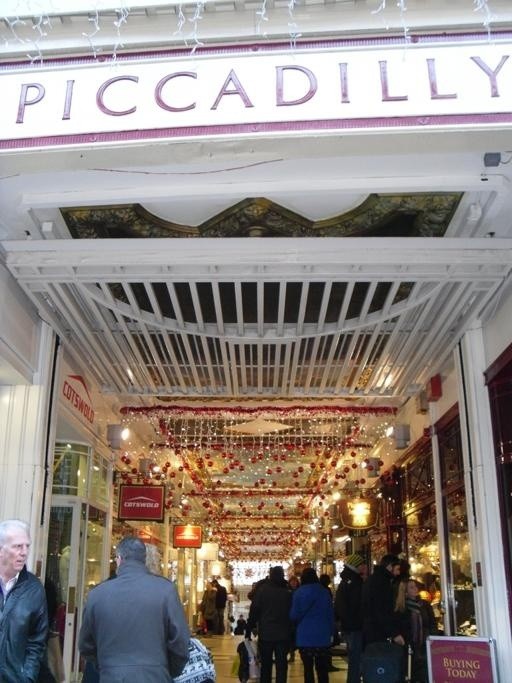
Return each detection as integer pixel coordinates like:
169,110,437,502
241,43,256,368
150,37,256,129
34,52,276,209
364,643,404,683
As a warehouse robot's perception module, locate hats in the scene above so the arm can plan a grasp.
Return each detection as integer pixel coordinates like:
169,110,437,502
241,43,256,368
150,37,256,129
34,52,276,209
344,554,363,567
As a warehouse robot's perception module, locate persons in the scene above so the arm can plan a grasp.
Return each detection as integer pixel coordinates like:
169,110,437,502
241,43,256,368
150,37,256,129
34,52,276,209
76,535,192,683
0,519,50,683
198,553,474,682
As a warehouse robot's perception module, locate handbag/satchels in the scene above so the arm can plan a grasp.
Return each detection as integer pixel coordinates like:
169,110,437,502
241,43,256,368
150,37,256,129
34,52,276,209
236,640,263,683
172,637,215,683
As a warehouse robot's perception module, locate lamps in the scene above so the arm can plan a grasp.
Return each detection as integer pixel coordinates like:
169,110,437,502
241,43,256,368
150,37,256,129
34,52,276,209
140,459,160,478
107,424,129,450
361,457,380,477
386,424,410,448
